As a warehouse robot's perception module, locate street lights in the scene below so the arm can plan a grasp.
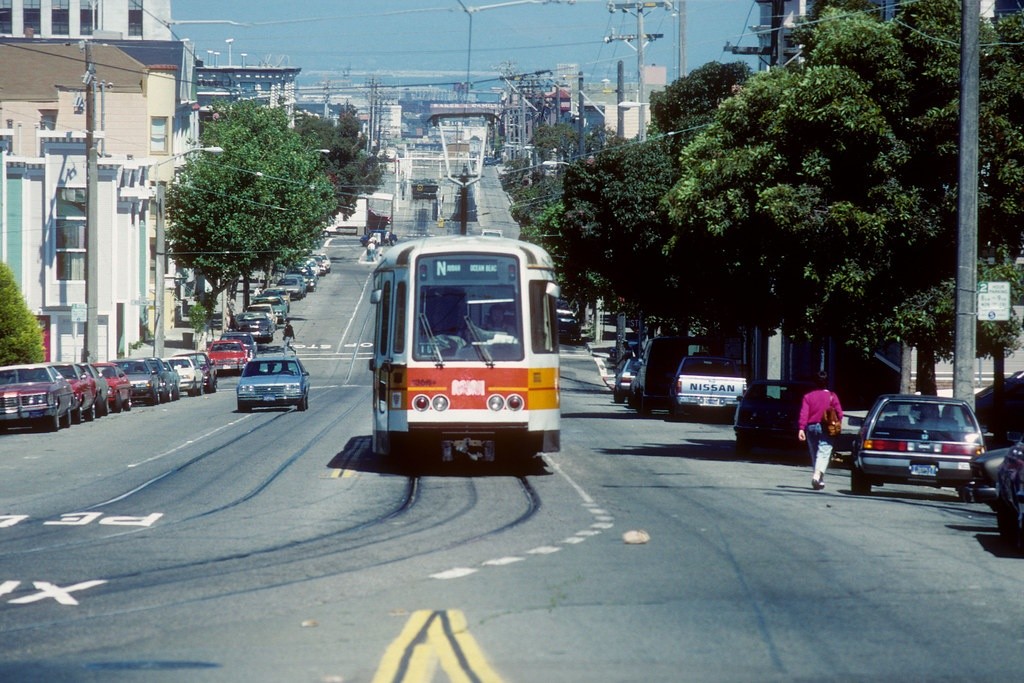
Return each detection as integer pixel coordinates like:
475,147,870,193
214,52,220,67
240,53,247,67
206,50,213,65
154,146,224,358
225,38,234,67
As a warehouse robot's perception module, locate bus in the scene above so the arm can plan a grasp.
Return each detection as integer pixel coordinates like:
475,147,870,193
368,237,564,469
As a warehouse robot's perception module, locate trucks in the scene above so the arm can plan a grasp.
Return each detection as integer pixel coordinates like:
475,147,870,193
335,225,359,236
320,220,337,238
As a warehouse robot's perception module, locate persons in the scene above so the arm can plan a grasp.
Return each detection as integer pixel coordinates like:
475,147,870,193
283,321,297,355
798,371,843,489
484,305,516,338
366,234,377,262
617,341,633,370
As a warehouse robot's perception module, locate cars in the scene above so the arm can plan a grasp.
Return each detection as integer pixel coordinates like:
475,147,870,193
231,304,279,343
733,370,1024,553
555,299,583,345
252,288,292,321
0,363,131,432
111,358,180,406
613,326,748,417
235,356,312,413
359,229,398,246
484,156,493,166
205,332,259,373
277,253,330,301
165,353,217,397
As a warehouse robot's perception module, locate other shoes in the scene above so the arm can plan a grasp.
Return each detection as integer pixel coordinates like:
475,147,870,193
819,483,824,489
812,479,820,490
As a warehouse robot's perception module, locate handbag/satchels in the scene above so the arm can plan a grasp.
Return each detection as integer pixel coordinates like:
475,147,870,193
822,392,842,436
